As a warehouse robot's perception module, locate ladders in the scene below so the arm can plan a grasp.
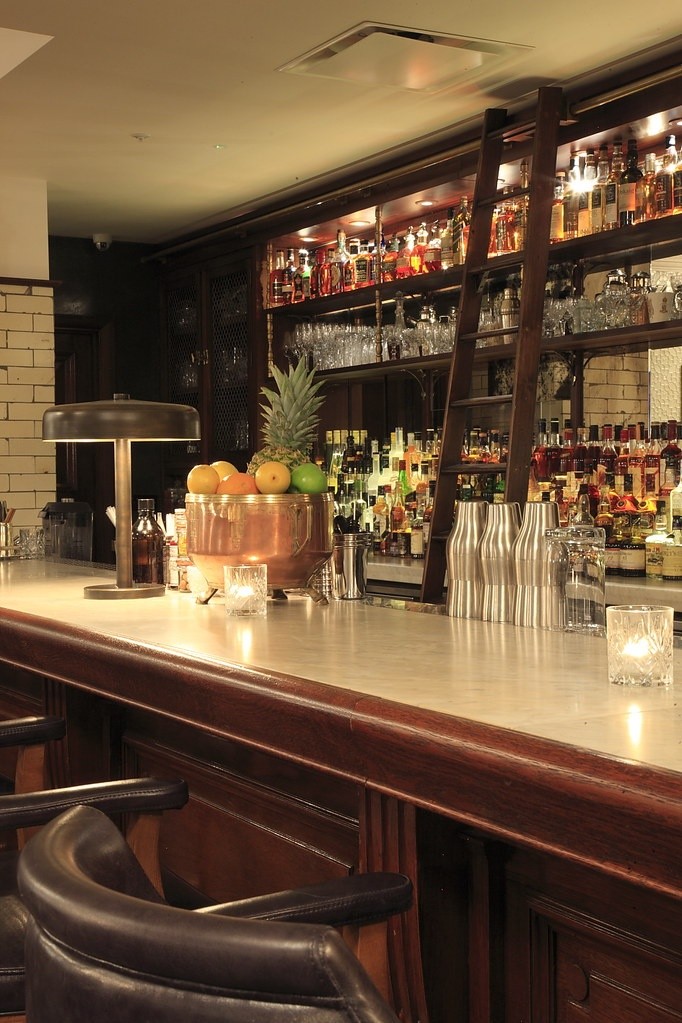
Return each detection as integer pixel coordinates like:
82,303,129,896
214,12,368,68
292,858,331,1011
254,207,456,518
418,86,562,606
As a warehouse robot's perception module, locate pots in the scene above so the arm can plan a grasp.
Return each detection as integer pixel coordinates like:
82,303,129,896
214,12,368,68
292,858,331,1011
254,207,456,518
184,493,335,606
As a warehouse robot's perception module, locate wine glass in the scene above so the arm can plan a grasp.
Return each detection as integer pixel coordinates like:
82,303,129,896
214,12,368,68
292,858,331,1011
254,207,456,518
283,296,682,369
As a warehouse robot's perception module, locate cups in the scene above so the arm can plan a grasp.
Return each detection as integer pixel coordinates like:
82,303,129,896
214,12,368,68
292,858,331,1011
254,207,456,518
0,523,14,560
544,527,605,632
606,604,673,689
647,291,674,324
225,564,267,618
20,529,45,561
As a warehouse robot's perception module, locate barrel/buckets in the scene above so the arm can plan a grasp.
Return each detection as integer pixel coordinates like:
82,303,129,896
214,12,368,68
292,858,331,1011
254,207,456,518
334,534,371,599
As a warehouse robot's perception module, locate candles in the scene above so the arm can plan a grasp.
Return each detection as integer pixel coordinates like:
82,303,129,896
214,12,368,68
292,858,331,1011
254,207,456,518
224,564,268,616
606,603,675,688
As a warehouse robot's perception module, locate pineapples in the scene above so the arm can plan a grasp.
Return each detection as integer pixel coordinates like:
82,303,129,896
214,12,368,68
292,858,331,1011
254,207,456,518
247,355,327,476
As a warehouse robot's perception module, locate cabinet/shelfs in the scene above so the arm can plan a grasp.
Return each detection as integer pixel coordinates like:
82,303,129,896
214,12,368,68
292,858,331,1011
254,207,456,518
261,64,682,384
155,248,263,487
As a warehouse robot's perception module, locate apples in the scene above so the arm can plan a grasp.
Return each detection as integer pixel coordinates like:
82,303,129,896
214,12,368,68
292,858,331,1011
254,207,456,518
287,464,327,494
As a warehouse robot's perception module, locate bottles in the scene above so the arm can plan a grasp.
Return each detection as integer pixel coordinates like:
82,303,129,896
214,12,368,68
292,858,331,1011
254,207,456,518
321,415,682,583
267,135,681,302
132,500,187,593
445,500,562,630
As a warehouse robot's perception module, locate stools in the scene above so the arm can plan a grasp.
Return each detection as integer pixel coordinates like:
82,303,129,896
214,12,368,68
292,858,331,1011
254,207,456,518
17,798,412,1023
0,714,190,1014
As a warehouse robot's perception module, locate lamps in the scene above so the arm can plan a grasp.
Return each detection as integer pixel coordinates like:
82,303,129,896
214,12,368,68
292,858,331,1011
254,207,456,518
41,392,202,603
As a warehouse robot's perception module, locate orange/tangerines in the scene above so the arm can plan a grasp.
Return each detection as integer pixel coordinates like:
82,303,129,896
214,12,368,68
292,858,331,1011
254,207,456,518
187,461,290,496
